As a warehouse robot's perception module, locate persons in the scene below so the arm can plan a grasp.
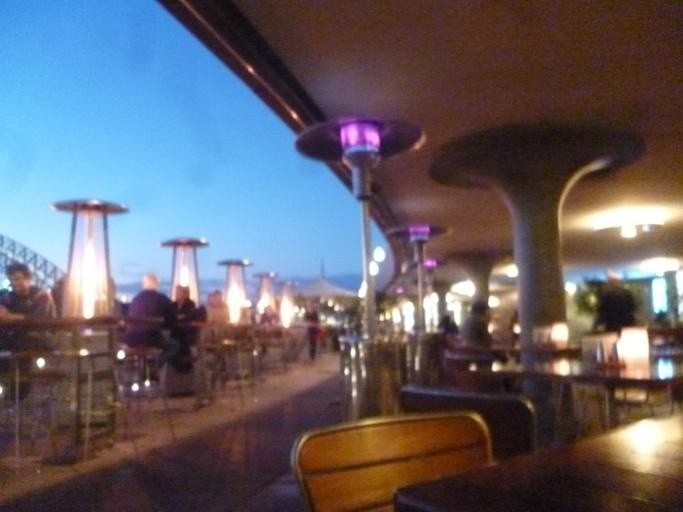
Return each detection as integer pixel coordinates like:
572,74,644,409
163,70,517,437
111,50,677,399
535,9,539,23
591,273,637,331
300,299,361,359
1,264,67,401
238,305,279,356
458,302,493,347
114,273,230,399
437,316,459,334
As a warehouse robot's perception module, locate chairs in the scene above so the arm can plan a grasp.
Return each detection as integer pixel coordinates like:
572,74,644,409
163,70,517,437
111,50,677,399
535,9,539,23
0,312,262,473
289,346,586,512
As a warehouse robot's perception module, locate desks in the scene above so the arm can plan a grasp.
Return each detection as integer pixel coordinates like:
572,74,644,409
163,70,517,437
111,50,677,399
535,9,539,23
391,350,683,511
